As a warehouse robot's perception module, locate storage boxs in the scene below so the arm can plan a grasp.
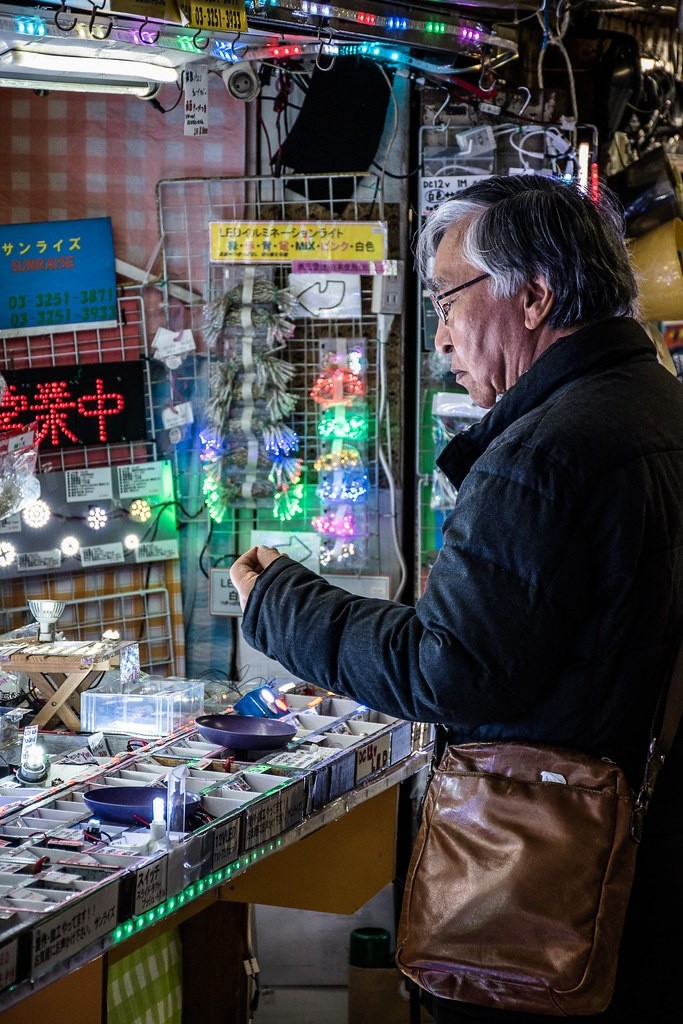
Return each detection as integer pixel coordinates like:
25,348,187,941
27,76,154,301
82,677,203,737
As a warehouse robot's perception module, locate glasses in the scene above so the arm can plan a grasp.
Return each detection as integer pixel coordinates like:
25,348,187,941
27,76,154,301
432,265,508,325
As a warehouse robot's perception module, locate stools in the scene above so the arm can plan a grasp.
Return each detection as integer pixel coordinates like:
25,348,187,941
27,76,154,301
0,640,138,731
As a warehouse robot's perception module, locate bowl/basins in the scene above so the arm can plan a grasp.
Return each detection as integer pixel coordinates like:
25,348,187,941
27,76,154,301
82,787,202,826
194,714,297,751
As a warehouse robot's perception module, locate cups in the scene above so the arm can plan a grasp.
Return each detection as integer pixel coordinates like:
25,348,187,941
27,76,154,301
349,926,392,969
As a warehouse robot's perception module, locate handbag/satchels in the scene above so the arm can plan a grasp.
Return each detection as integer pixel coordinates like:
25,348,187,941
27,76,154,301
395,739,644,1016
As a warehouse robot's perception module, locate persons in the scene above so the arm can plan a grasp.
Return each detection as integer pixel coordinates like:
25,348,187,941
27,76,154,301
229,176,682,1023
